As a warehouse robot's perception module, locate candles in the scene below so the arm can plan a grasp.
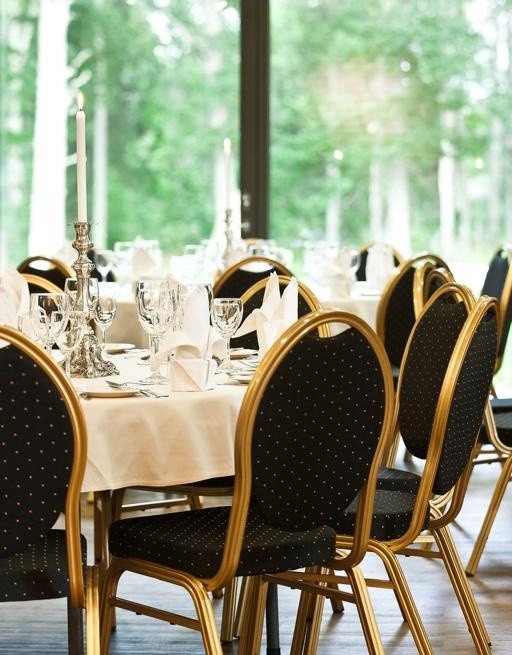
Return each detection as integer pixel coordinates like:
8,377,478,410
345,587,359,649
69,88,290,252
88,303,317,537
75,111,86,223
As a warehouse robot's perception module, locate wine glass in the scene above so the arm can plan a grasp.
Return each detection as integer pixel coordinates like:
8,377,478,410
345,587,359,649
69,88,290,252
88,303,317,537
135,288,175,385
93,240,362,296
211,297,244,376
16,275,116,384
